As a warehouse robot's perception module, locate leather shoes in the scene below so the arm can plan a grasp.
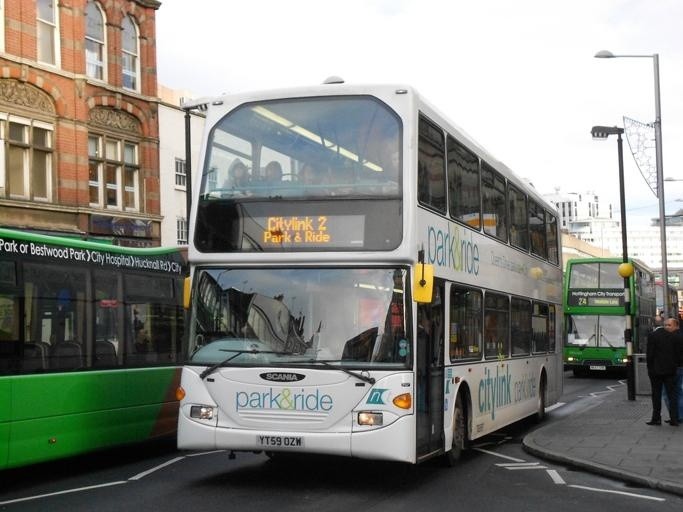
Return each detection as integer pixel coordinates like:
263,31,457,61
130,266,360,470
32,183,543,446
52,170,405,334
646,421,661,425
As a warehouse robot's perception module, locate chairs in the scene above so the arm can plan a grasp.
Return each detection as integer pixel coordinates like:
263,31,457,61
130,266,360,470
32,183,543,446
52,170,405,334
22,339,118,370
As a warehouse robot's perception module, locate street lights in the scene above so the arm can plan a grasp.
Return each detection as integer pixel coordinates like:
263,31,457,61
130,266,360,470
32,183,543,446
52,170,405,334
591,126,637,403
182,98,210,244
594,51,669,319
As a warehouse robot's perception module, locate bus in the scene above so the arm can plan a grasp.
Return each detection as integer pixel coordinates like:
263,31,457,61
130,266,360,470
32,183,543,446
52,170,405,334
0,228,188,471
655,279,679,329
563,258,656,377
175,83,563,467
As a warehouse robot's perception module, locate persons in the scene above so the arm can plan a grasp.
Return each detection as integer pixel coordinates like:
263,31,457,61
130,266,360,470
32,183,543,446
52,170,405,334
663,317,683,425
646,315,679,426
221,151,401,197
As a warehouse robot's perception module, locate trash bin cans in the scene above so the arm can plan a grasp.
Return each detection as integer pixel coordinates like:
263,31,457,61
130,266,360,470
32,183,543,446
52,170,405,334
633,353,654,396
